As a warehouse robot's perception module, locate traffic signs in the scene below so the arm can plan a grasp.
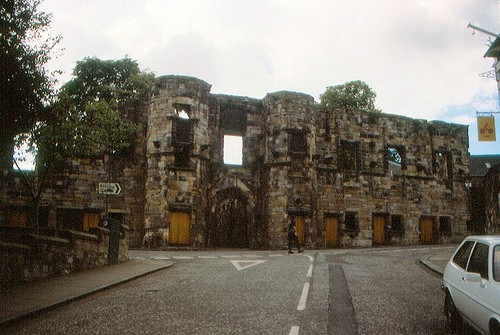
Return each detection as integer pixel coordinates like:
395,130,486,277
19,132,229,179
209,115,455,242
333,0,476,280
99,183,121,195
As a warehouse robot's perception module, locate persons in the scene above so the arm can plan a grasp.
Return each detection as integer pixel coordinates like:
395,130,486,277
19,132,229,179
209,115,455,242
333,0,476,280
287,214,305,254
384,225,395,247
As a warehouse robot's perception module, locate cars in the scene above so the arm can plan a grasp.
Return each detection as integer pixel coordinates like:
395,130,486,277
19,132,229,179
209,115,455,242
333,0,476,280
441,235,500,335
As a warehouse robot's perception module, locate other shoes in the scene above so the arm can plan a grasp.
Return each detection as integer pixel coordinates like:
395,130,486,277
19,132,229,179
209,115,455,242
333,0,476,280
298,250,304,253
288,251,294,254
